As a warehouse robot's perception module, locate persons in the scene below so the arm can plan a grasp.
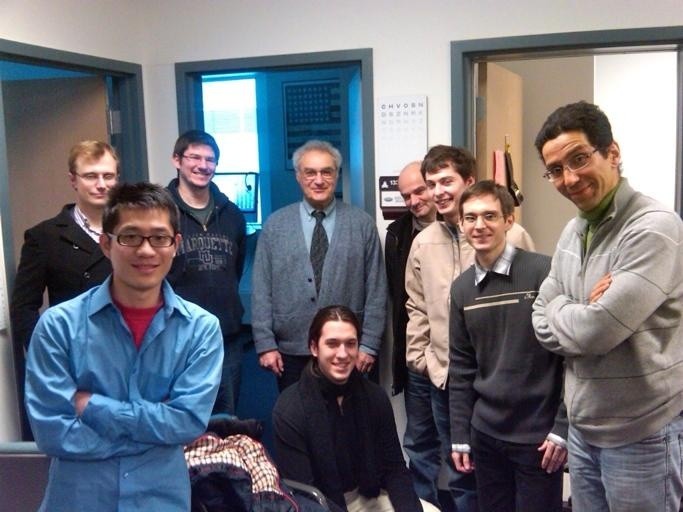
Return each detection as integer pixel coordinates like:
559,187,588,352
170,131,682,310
9,138,121,353
249,138,390,396
271,303,444,512
159,130,246,421
529,97,683,511
402,143,537,510
21,178,226,510
382,158,448,512
444,179,572,510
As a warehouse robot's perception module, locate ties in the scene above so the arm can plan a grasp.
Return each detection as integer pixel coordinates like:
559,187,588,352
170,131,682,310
311,209,328,294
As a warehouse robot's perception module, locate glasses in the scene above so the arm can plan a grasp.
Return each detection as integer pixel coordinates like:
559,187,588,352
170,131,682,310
543,144,608,183
106,230,178,249
462,214,509,222
72,171,117,180
298,169,337,179
181,154,218,164
